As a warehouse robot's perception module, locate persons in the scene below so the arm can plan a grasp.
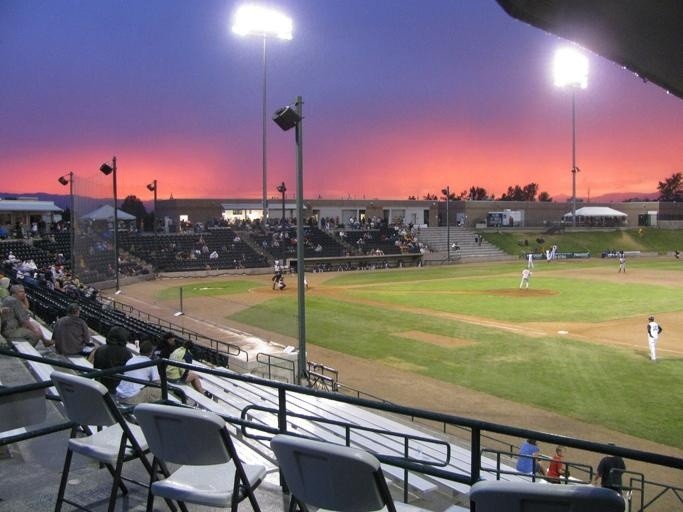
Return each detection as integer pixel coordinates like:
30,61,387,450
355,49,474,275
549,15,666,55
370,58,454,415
552,243,557,252
515,437,546,476
592,442,626,495
617,255,626,273
546,247,556,263
673,247,681,260
0,273,213,421
543,445,571,484
518,267,532,291
545,249,549,259
1,212,428,272
646,315,663,360
270,272,287,291
451,242,460,250
526,253,535,269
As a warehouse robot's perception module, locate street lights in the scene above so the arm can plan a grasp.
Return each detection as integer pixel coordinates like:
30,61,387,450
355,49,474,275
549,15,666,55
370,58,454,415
550,46,589,232
275,181,288,268
229,0,296,226
440,184,453,261
58,169,76,279
272,88,312,384
96,154,119,295
145,177,158,227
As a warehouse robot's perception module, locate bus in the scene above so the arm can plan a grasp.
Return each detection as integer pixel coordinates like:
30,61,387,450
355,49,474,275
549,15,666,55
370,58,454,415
485,208,522,227
